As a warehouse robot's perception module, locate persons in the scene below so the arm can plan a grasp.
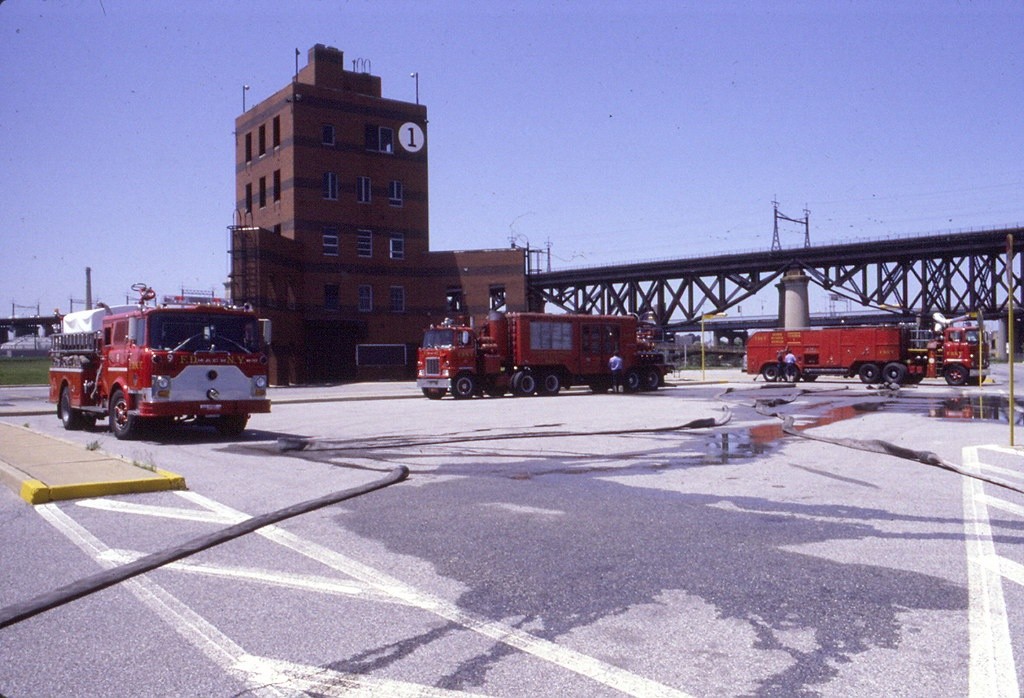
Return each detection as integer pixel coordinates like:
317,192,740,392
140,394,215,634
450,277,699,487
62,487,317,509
608,349,624,393
776,346,796,383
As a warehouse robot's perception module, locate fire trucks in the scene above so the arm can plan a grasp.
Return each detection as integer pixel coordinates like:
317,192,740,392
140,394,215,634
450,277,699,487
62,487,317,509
415,312,675,401
48,300,271,439
745,327,989,386
